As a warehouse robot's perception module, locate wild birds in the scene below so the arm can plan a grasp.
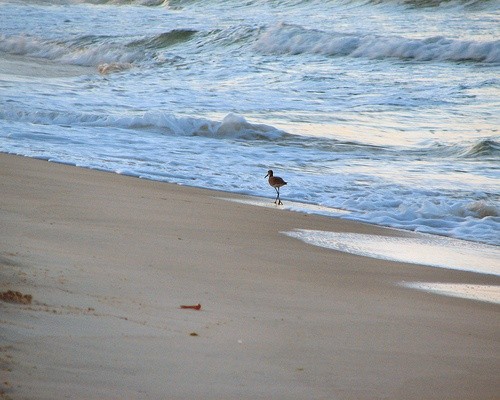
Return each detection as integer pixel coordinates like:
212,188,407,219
264,170,288,205
